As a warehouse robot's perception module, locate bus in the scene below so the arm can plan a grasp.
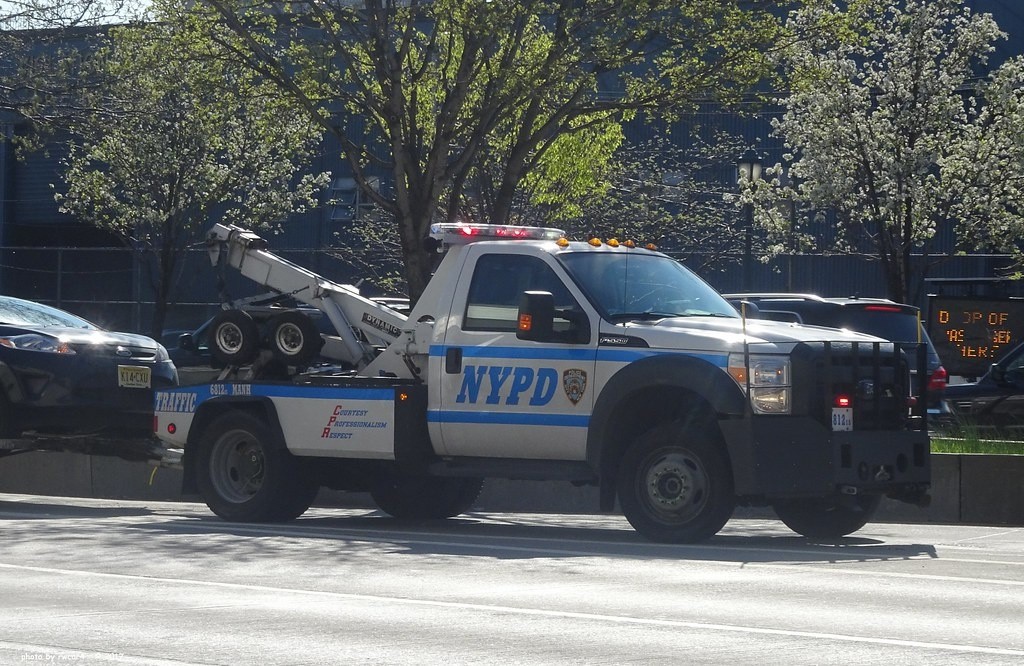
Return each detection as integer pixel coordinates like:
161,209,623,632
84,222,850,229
919,277,1023,383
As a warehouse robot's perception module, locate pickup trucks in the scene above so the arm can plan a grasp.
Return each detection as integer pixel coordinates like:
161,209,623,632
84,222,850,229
150,221,934,545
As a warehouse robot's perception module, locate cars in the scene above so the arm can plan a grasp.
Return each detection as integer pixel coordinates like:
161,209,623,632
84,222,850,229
947,343,1024,426
0,294,179,416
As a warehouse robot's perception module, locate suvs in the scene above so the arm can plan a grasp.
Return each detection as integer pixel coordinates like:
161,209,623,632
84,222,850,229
720,292,954,429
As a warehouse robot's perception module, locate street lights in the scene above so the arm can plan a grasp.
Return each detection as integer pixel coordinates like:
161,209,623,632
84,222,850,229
736,147,764,293
